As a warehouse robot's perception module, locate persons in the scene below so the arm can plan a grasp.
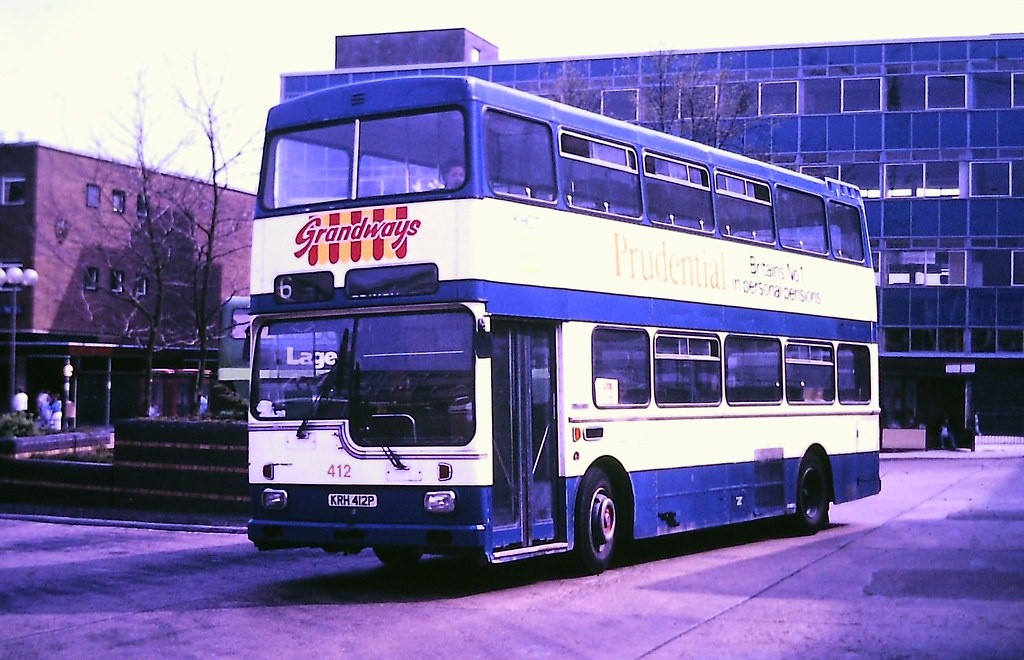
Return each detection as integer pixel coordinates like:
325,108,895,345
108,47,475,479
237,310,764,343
939,417,956,450
432,158,466,192
11,385,76,432
970,412,984,452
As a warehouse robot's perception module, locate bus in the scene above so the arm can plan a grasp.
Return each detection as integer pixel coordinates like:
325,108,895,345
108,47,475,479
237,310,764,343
228,74,882,577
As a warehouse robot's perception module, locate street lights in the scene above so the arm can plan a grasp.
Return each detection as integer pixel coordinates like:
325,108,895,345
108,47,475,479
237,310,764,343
0,267,39,399
